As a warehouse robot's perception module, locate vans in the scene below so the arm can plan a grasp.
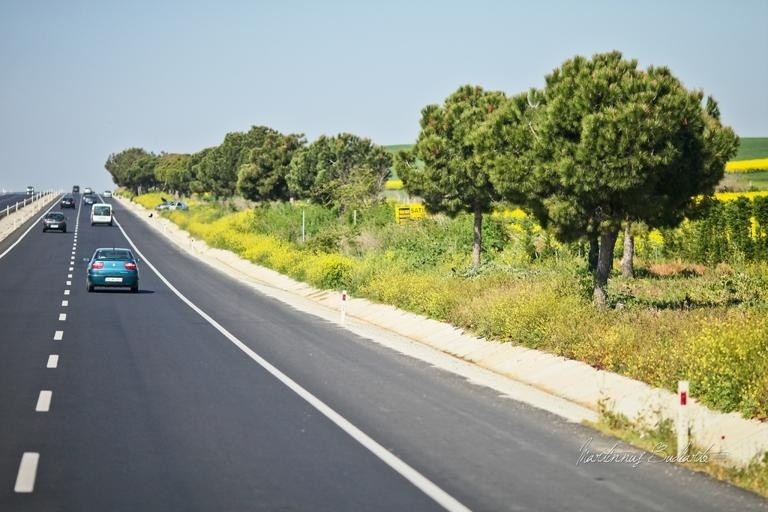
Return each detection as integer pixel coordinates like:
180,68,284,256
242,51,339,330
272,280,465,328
89,203,113,225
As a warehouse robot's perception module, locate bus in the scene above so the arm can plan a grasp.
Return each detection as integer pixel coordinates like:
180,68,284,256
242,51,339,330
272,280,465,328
72,185,80,195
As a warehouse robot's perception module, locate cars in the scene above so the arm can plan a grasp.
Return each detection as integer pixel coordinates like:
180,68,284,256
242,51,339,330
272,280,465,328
42,211,68,233
103,190,113,198
82,187,97,205
156,197,187,211
26,185,34,192
60,197,76,210
83,247,140,294
